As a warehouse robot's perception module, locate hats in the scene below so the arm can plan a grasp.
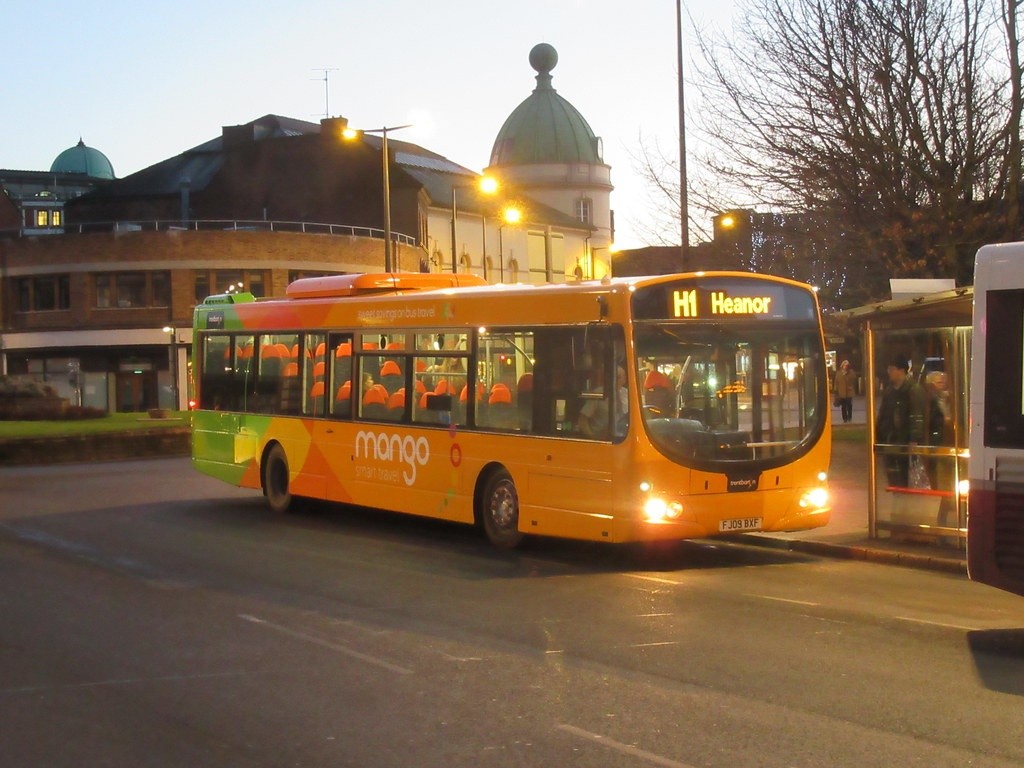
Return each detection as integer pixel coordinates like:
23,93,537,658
890,355,908,369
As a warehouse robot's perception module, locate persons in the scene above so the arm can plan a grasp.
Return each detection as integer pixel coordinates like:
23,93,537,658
577,366,628,434
362,355,467,399
834,360,856,423
418,334,467,389
876,359,953,490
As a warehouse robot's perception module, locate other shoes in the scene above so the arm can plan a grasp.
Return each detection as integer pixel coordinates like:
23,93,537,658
844,419,848,423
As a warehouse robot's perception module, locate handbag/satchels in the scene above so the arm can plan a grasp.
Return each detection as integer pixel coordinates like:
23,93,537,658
833,398,841,407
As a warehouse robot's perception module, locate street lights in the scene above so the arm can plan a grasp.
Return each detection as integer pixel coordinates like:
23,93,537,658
339,126,392,272
451,176,499,273
482,207,521,281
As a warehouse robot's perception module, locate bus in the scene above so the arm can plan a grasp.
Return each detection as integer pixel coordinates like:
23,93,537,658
965,241,1024,597
190,272,834,551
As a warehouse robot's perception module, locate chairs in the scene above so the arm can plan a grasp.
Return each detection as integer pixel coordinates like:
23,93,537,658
518,373,534,389
488,383,513,406
459,383,485,416
224,343,457,417
644,370,674,403
